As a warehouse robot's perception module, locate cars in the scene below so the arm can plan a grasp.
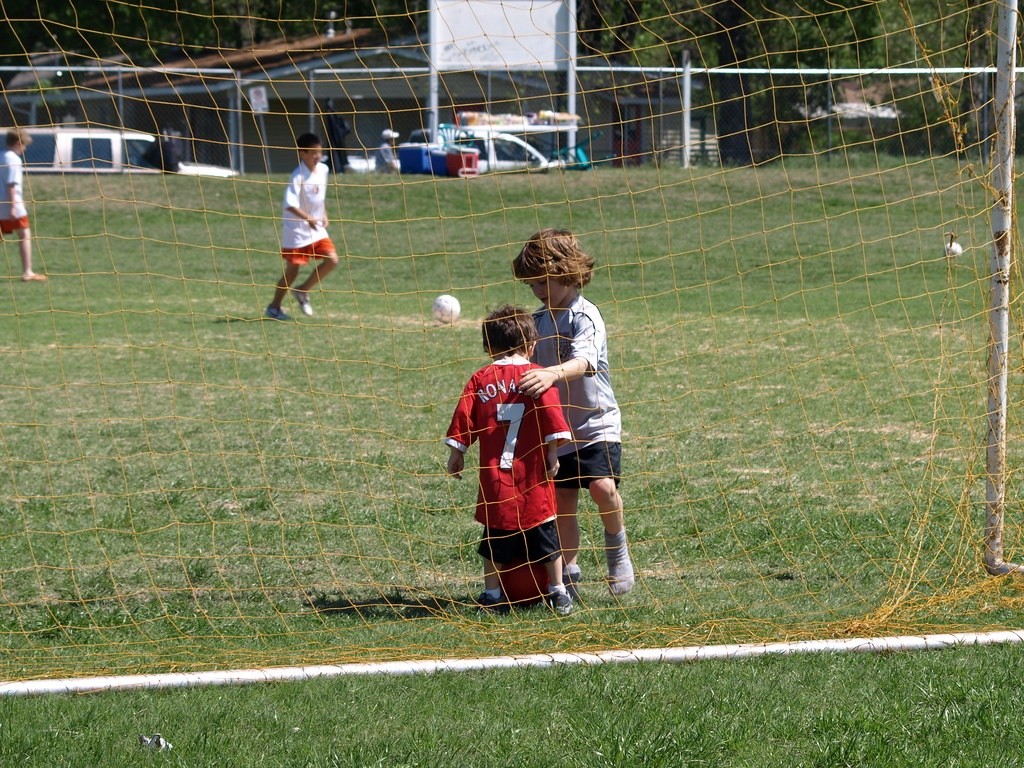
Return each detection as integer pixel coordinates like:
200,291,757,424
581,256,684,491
408,127,550,173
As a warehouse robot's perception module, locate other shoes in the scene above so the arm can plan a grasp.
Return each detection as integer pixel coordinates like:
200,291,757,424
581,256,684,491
547,591,572,616
22,272,47,282
476,593,507,615
294,287,313,317
265,304,290,320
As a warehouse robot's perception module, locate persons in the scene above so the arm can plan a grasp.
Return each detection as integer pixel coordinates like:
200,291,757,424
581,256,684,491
264,133,340,321
376,129,400,175
447,308,580,615
511,228,634,601
0,129,48,282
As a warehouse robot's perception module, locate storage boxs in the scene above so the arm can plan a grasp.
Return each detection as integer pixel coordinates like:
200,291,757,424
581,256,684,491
398,143,479,176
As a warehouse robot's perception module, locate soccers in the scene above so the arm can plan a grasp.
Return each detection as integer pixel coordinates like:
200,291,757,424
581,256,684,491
431,294,461,323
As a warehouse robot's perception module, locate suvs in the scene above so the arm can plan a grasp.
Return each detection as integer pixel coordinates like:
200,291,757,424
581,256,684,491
0,125,238,175
